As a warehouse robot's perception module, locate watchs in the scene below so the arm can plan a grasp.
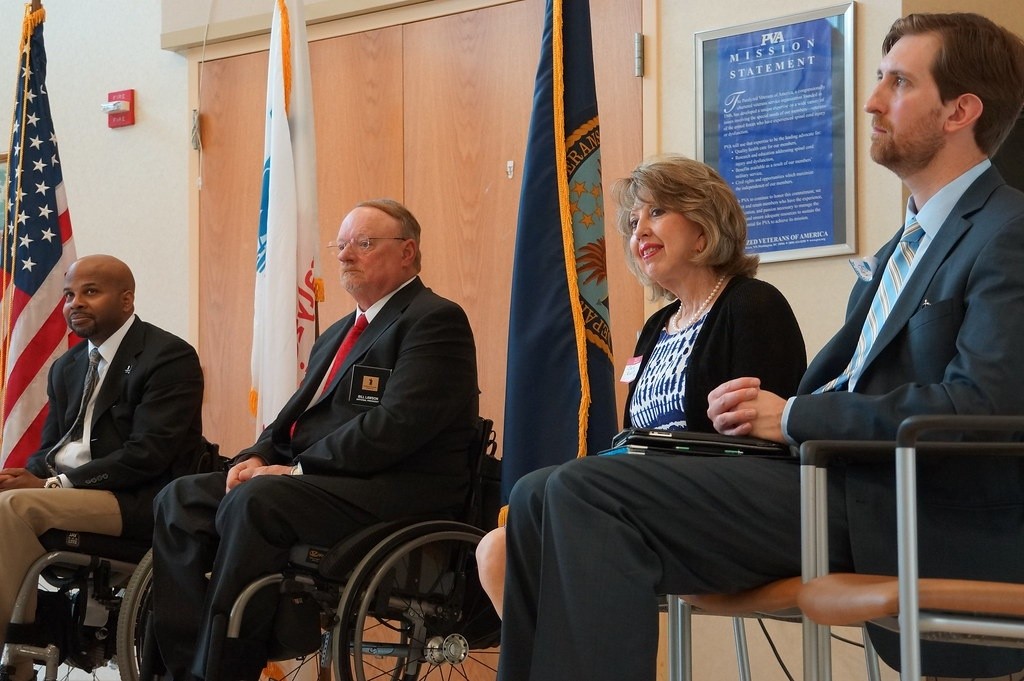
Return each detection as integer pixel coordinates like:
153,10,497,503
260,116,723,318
291,465,298,475
44,477,62,488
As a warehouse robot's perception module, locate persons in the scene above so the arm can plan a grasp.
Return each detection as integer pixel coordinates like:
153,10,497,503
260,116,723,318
475,12,1024,681
152,200,482,681
0,254,205,681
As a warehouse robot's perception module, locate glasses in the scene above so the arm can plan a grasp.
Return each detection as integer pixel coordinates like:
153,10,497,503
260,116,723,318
327,236,406,251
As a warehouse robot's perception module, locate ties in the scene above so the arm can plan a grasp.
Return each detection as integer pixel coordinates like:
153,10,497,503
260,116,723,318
290,313,370,441
70,348,103,444
809,216,924,397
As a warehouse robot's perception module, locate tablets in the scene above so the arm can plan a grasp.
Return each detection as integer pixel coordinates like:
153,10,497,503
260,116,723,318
614,429,783,452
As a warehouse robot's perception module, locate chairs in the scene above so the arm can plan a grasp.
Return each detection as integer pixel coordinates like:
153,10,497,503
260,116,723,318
657,415,1024,681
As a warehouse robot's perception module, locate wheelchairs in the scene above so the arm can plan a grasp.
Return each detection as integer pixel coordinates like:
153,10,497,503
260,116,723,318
0,432,219,681
117,412,507,681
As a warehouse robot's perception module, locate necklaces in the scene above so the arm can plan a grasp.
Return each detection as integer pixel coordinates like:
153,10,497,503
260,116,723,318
672,273,728,332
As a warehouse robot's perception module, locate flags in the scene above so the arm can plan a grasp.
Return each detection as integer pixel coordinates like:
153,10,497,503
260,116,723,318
498,0,618,525
0,3,86,468
247,0,326,443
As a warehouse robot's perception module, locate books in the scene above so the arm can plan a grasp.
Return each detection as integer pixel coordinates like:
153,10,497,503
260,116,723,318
596,427,791,457
